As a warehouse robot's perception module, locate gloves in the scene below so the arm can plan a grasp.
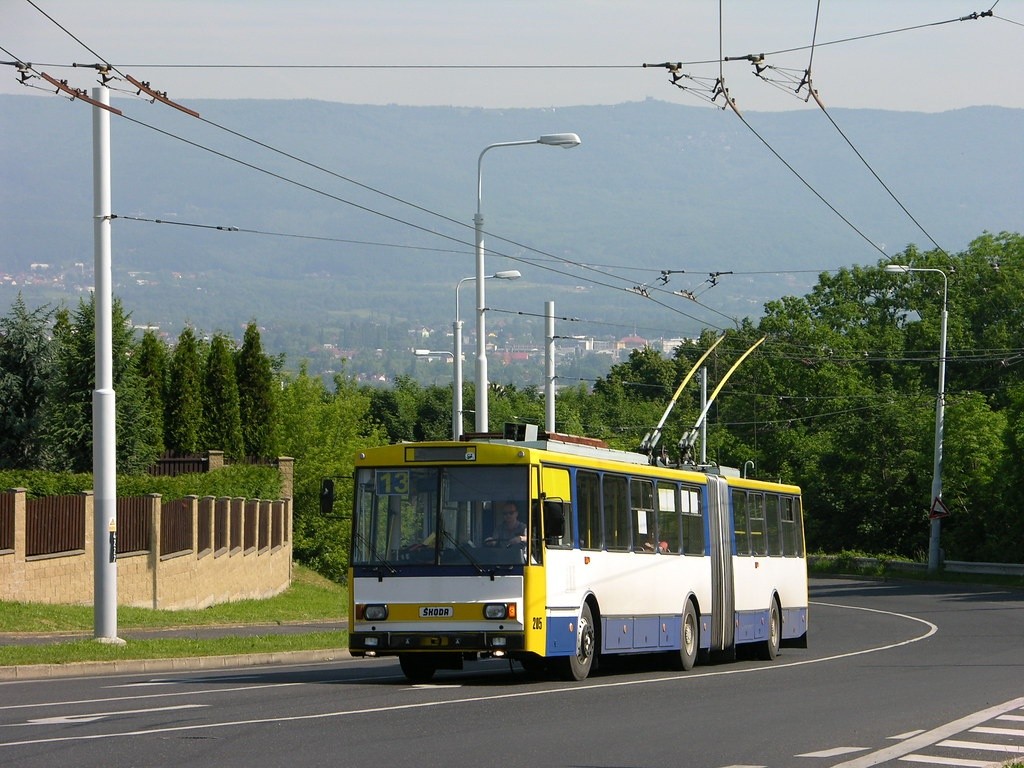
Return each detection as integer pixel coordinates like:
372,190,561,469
506,536,521,547
485,537,497,547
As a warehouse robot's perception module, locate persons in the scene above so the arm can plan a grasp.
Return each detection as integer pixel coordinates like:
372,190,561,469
482,500,528,548
644,522,668,553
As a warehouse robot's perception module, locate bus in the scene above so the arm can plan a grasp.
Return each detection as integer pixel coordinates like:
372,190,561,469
320,422,808,686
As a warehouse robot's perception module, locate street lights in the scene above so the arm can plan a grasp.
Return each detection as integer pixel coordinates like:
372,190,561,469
452,270,521,440
475,133,582,432
884,264,948,572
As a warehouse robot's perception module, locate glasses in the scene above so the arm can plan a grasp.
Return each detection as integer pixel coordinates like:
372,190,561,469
503,511,516,515
